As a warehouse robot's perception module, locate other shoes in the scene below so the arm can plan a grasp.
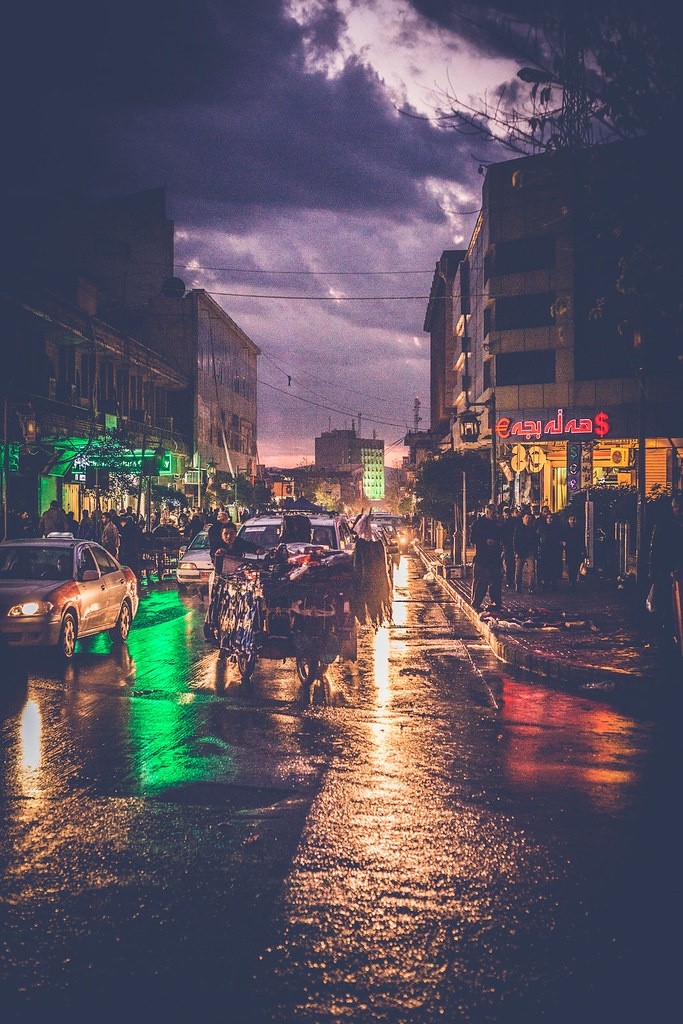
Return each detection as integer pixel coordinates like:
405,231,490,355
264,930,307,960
474,607,483,612
495,605,507,612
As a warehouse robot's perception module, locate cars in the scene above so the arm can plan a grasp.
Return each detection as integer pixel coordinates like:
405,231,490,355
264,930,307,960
0,531,139,661
176,508,406,600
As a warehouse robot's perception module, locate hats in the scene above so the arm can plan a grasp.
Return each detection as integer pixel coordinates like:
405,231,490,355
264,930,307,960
502,506,509,511
216,512,228,520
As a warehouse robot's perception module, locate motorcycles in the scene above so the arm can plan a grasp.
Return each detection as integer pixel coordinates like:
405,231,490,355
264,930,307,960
204,540,394,687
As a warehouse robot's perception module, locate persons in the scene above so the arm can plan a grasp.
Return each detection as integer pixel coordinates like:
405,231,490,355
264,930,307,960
0,493,683,661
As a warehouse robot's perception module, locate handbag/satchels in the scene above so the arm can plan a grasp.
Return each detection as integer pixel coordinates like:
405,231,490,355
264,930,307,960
646,584,657,613
